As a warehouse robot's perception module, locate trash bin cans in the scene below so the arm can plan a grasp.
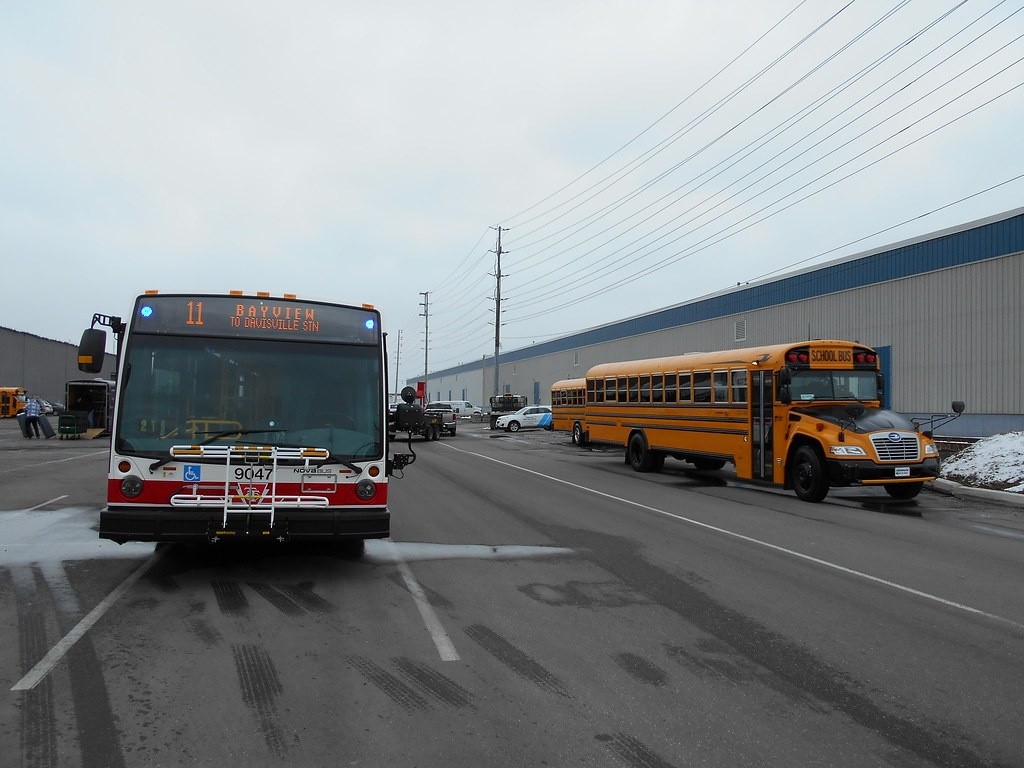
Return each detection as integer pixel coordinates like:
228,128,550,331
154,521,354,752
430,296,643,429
490,414,508,430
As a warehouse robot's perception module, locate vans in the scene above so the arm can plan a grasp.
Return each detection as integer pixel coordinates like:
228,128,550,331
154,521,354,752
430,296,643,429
432,400,482,418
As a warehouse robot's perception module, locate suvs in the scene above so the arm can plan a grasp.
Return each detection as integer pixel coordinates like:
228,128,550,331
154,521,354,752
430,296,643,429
423,403,457,436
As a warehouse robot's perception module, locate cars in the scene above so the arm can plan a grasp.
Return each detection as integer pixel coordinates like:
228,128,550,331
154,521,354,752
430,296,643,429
35,397,67,417
496,406,552,432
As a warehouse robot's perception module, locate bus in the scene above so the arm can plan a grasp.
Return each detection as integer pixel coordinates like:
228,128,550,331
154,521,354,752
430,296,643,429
0,387,32,418
585,338,967,504
75,287,418,542
550,378,586,446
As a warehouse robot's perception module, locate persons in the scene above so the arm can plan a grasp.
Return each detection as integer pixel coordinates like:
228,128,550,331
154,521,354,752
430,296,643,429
23,398,41,440
480,410,484,423
